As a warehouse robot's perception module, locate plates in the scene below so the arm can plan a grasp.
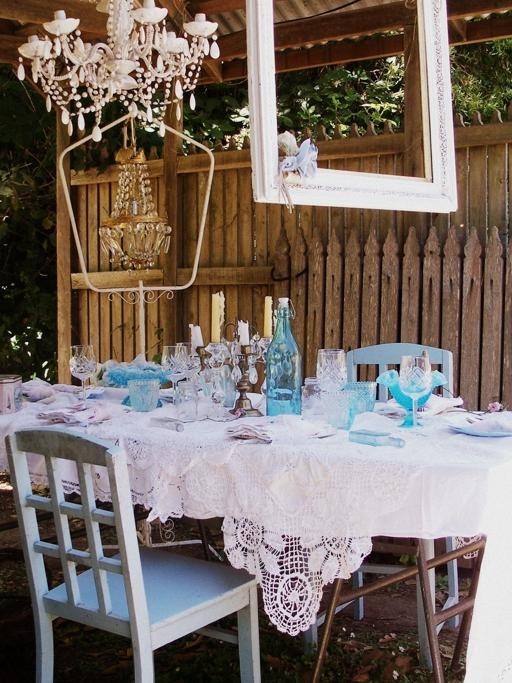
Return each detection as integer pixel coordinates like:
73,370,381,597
387,392,463,416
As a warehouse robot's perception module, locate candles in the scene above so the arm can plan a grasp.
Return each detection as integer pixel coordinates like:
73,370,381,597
263,295,273,337
187,321,202,355
238,319,249,345
211,292,220,343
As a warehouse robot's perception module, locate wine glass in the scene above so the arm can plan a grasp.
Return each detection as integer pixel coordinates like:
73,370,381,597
317,348,346,393
161,346,202,408
69,345,96,405
376,356,449,428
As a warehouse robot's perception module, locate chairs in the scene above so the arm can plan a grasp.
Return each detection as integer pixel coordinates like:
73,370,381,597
2,428,264,683
303,341,461,648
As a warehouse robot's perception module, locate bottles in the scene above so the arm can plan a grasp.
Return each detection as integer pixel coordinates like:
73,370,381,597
175,382,200,422
303,378,328,428
350,429,406,447
266,297,302,415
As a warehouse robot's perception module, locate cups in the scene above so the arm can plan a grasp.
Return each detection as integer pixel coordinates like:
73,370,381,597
347,382,376,426
127,379,160,412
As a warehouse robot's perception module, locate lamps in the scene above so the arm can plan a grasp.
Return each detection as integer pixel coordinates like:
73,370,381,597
55,110,215,362
16,0,221,141
